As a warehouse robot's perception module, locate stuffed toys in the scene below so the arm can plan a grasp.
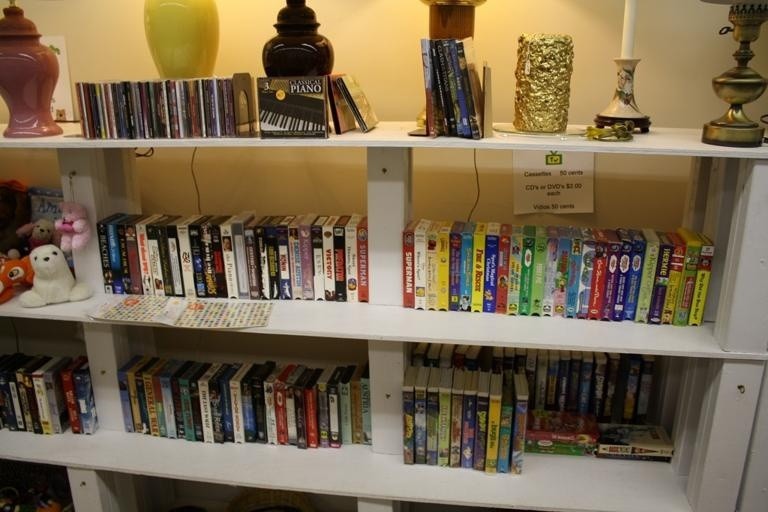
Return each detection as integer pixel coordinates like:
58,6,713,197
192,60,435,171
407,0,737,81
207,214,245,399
7,218,52,259
19,244,94,308
0,255,34,305
53,201,91,251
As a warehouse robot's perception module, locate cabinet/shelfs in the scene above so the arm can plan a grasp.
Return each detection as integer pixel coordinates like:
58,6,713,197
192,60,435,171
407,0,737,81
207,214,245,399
0,120,766,510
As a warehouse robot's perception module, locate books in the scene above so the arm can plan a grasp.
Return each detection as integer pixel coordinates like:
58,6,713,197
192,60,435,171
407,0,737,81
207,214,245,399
116,353,372,450
400,343,675,475
96,209,367,302
420,37,482,138
0,352,99,436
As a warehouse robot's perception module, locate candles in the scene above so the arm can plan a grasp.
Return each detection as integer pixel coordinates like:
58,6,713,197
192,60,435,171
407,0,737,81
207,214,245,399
620,1,638,58
